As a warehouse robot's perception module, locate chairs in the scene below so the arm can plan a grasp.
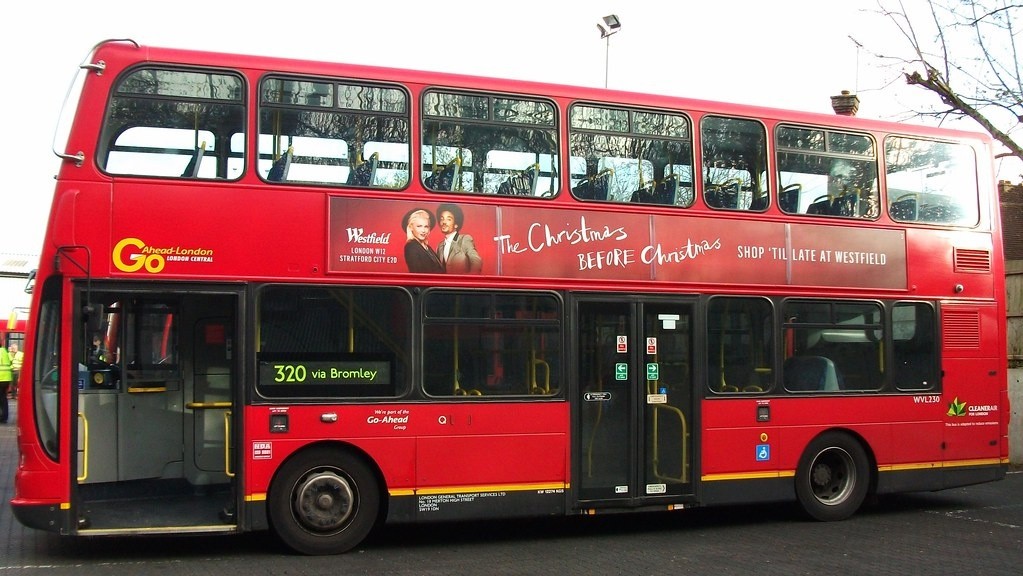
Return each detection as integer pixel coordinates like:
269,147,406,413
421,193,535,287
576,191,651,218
180,141,206,178
806,193,834,216
706,186,719,207
424,165,444,189
592,168,615,202
438,158,462,190
518,163,539,196
346,152,379,186
268,146,293,181
631,180,656,204
782,183,802,213
652,172,681,205
783,354,840,391
720,178,741,209
749,190,768,209
890,193,918,221
572,176,593,200
837,188,860,216
497,175,518,194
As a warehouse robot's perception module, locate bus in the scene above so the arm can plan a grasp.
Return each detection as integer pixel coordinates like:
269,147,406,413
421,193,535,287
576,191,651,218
0,34,1009,553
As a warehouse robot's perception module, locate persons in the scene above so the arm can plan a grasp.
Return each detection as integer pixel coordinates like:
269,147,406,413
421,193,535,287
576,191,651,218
437,205,482,275
7,342,28,400
0,345,11,422
93,336,105,361
401,209,445,274
128,332,173,367
